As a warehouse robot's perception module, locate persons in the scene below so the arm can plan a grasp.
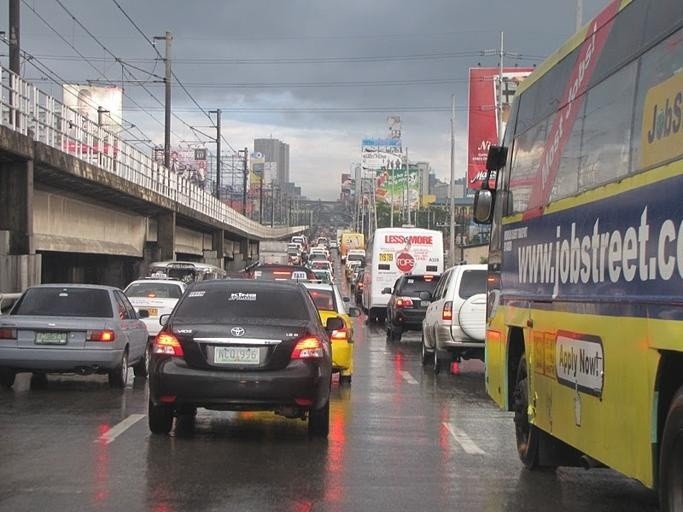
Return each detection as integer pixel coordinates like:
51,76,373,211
66,89,98,143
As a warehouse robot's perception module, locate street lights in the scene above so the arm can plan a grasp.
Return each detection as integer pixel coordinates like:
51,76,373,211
359,147,417,233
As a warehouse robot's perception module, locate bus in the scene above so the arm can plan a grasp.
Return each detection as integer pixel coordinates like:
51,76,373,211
470,2,682,512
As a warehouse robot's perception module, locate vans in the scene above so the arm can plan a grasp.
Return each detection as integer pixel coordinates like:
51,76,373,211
359,229,445,325
148,261,229,287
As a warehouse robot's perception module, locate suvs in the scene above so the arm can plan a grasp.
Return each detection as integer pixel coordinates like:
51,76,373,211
420,264,489,376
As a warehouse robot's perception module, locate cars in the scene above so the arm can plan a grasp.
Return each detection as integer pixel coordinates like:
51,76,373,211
119,279,188,344
149,279,332,438
385,273,440,342
242,224,365,387
0,282,151,389
